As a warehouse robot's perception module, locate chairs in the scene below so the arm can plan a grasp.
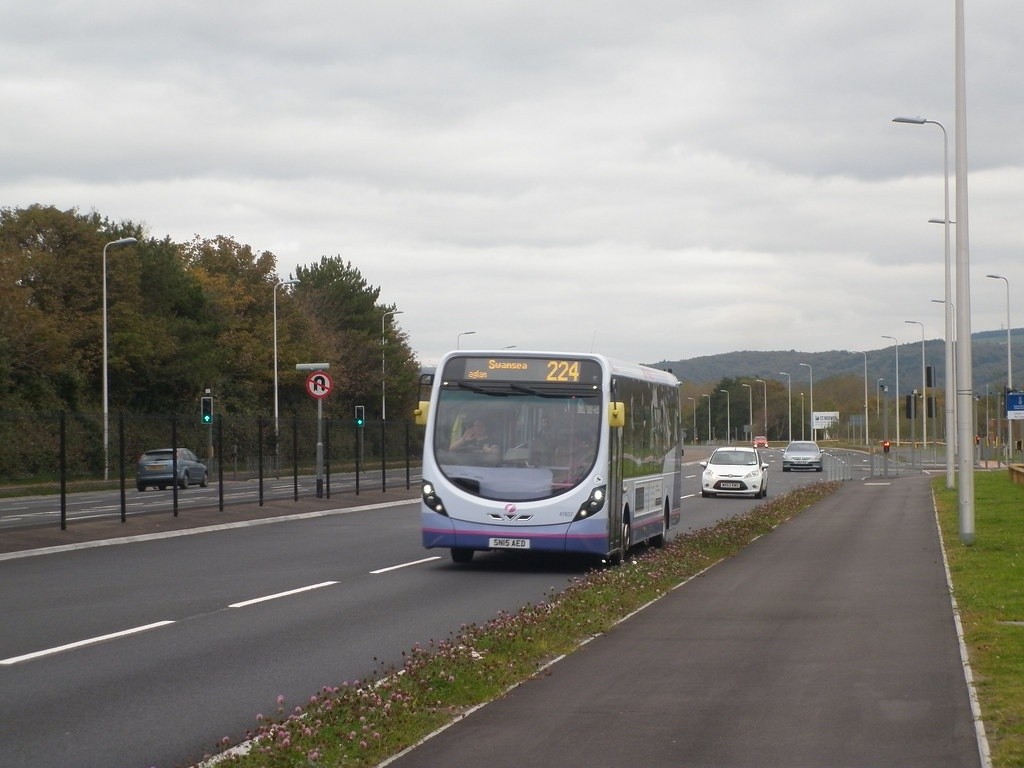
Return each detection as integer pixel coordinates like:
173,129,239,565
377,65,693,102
504,448,532,461
744,453,754,462
714,454,733,464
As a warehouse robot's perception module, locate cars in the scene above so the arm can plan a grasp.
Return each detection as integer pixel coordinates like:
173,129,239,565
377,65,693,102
700,447,770,499
753,435,769,449
780,440,826,472
135,446,208,491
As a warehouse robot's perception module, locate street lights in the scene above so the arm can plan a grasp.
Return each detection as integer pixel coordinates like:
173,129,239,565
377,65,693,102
853,350,868,445
688,398,696,445
931,298,958,448
720,389,731,445
877,378,883,415
295,363,329,498
880,335,900,447
102,236,137,480
273,278,301,457
702,394,711,445
986,273,1013,461
904,320,927,449
457,331,477,350
756,379,767,438
800,392,804,440
799,363,813,441
891,114,956,491
381,310,404,421
779,373,792,444
741,383,753,446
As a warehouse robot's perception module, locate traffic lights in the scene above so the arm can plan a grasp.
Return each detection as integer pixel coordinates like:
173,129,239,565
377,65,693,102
200,396,213,424
355,406,364,427
975,436,979,445
883,440,890,453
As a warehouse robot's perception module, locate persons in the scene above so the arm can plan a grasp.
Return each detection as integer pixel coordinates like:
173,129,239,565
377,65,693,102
447,416,501,454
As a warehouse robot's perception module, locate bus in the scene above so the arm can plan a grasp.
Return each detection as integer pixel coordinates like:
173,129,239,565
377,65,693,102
413,350,682,568
